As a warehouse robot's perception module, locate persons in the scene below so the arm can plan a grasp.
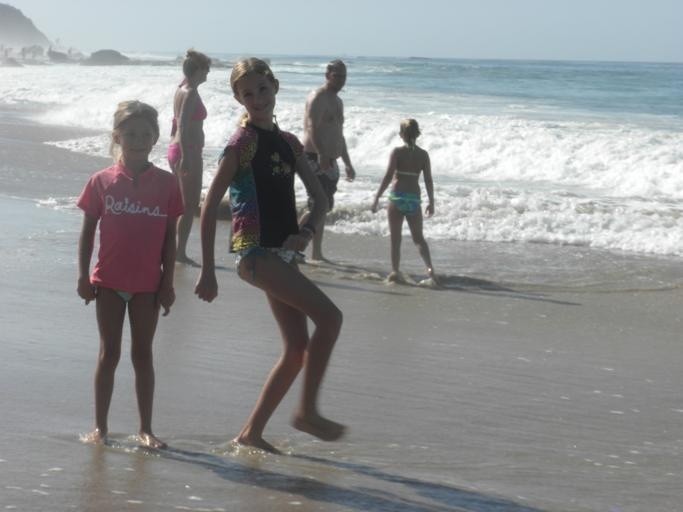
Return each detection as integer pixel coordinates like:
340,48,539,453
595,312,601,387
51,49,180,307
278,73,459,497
167,49,211,268
192,59,352,454
75,100,183,451
293,58,356,265
372,116,438,286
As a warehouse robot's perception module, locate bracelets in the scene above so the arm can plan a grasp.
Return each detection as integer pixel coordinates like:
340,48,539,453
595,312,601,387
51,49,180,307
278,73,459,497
297,223,319,237
